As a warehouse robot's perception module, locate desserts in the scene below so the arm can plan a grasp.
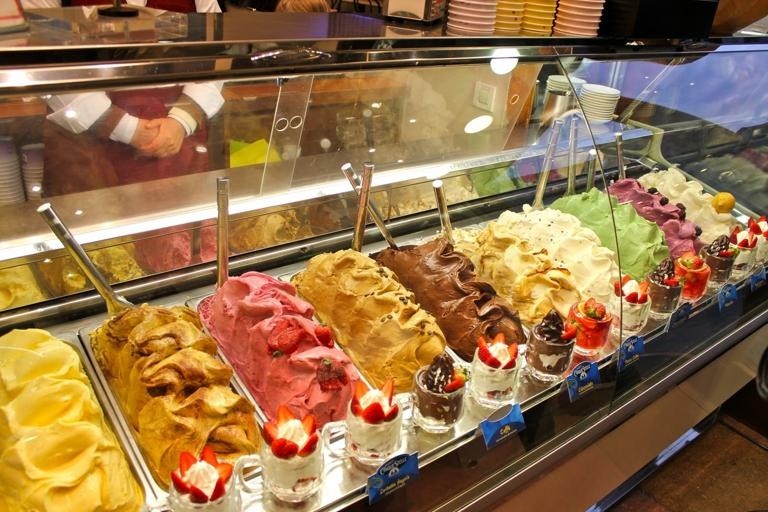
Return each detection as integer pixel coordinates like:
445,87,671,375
1,163,768,512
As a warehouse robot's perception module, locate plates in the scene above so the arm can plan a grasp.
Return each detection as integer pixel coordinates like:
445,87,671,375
444,0,606,36
543,73,620,126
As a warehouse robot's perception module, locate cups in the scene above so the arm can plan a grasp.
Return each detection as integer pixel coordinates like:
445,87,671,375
645,272,683,319
459,343,521,409
395,366,471,435
726,229,768,279
235,430,327,505
567,300,612,356
610,292,651,339
148,457,236,511
0,135,46,207
319,396,402,465
702,245,742,289
674,254,712,304
524,325,577,385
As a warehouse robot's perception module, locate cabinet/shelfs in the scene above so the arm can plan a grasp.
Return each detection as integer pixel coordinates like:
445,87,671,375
0,40,768,511
216,65,404,171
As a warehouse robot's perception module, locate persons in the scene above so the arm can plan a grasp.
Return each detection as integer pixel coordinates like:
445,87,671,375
274,0,333,14
12,0,222,198
